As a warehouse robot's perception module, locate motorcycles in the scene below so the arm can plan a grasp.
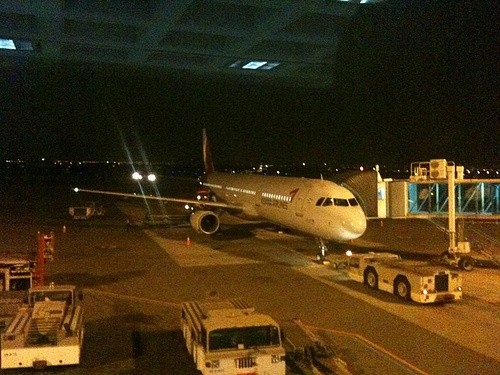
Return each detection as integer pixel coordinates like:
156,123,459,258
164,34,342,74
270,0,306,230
440,249,461,269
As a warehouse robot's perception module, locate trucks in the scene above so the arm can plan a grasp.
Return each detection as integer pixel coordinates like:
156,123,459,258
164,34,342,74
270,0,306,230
347,251,464,306
180,297,287,375
0,283,84,370
69,201,106,225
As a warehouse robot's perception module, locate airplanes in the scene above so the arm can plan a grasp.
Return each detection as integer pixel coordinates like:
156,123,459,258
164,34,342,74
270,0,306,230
70,128,367,265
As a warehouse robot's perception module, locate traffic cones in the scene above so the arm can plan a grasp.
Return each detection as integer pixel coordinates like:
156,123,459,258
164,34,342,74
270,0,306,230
63,225,67,233
185,236,191,247
